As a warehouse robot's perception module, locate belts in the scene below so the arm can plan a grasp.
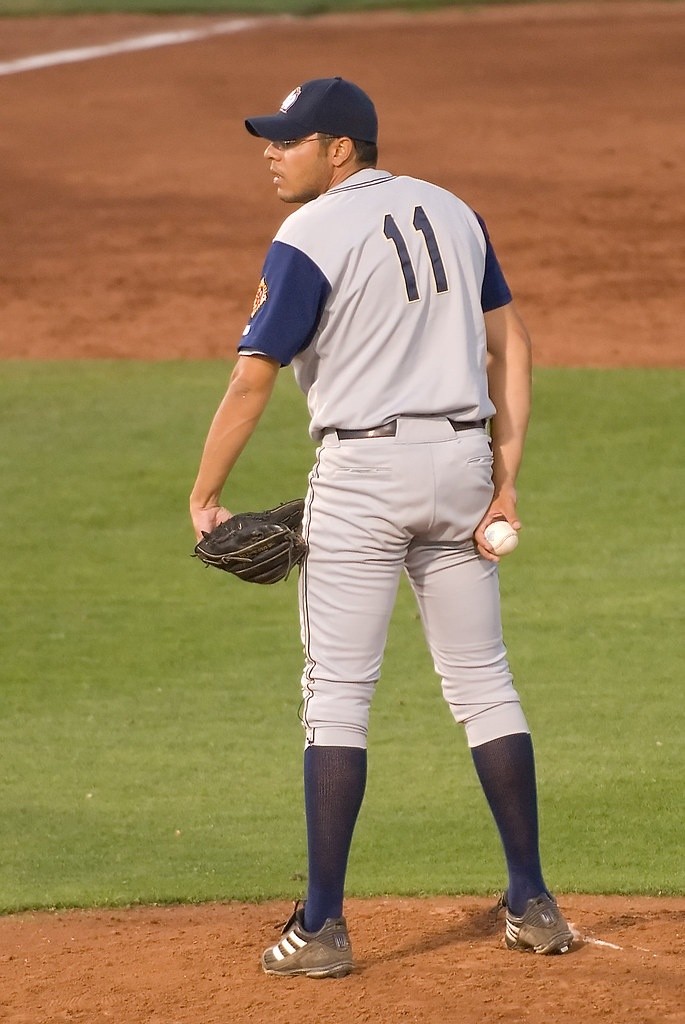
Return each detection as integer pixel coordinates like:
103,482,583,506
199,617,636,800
337,418,486,439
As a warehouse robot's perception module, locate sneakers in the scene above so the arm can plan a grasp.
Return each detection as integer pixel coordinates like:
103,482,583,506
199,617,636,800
262,899,353,978
489,889,574,953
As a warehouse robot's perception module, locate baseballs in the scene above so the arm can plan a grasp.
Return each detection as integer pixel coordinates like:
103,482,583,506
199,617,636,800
484,520,519,556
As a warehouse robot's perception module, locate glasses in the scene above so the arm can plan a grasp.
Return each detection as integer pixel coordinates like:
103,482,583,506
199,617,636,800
277,136,343,149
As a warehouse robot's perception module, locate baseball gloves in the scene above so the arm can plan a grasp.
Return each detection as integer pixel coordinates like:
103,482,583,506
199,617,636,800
194,498,309,585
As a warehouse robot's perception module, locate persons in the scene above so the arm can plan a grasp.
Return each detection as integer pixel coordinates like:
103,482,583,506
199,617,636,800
190,77,573,976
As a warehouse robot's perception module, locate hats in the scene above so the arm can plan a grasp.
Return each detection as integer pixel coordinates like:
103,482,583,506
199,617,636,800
245,77,379,143
